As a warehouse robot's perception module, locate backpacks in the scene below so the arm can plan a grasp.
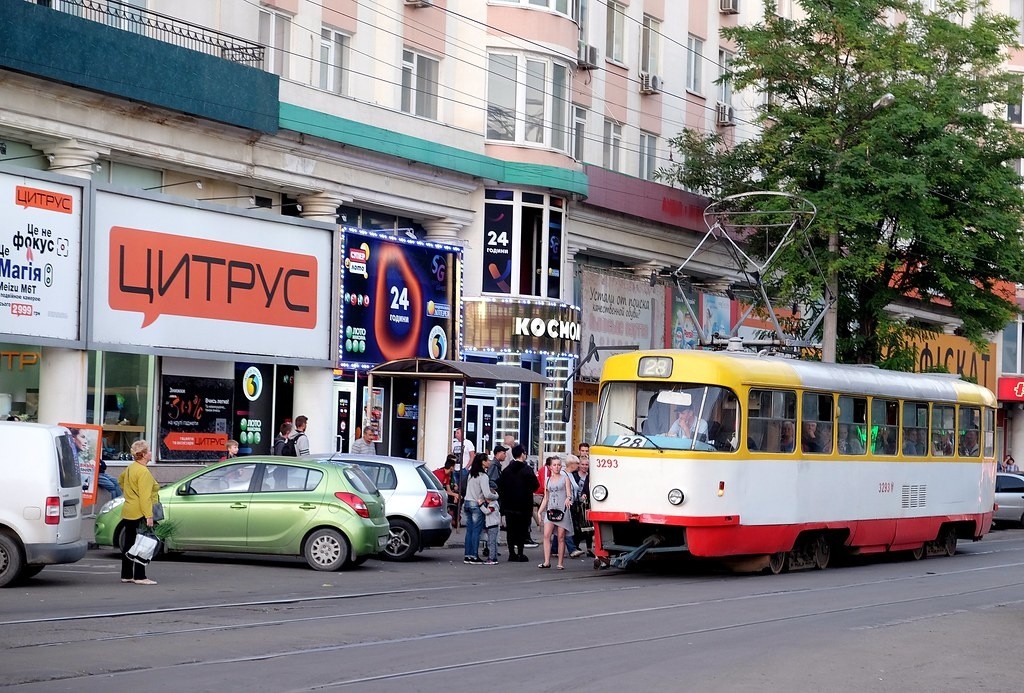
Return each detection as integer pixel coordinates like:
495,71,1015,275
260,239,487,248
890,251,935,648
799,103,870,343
282,434,304,456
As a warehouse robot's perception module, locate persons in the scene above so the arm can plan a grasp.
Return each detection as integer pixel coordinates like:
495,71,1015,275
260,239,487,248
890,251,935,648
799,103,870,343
433,428,595,570
997,454,1020,472
646,384,980,458
8,416,21,421
98,433,160,585
220,440,244,480
351,426,377,485
68,428,89,450
275,416,311,488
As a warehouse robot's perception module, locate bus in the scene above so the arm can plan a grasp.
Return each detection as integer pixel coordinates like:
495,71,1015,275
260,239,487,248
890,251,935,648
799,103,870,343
585,336,998,574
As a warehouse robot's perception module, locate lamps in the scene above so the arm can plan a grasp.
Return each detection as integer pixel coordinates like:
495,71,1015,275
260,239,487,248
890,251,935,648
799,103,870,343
41,162,102,172
439,239,472,249
372,228,417,240
0,142,55,163
245,203,302,211
300,213,347,222
143,180,203,190
612,266,797,315
197,195,255,204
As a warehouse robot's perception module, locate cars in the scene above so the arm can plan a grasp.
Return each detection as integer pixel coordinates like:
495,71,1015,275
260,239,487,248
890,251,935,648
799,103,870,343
93,455,390,572
993,473,1024,528
222,455,453,560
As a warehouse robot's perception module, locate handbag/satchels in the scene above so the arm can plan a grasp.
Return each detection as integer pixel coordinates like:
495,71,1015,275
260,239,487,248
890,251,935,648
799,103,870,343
547,509,564,521
151,503,164,521
125,527,158,565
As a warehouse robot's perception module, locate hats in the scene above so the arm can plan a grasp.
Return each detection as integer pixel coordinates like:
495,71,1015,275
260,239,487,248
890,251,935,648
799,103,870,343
674,404,694,412
494,445,509,455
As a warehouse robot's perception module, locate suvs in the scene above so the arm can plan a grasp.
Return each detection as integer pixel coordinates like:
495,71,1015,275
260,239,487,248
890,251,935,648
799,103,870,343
0,421,89,588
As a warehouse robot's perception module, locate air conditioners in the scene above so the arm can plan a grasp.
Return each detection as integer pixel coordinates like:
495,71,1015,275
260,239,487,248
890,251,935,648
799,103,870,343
718,104,736,123
719,0,740,14
579,44,599,70
643,73,661,94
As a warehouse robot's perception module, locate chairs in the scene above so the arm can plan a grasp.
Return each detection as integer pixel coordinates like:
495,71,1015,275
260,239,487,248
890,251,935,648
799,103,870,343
707,421,722,439
848,435,925,456
261,467,288,491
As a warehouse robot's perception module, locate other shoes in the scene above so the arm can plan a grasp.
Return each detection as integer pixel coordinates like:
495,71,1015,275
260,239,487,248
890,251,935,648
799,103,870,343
482,548,501,557
570,551,585,559
122,579,134,582
557,565,564,570
509,553,529,561
463,556,484,564
483,558,498,564
552,554,567,557
135,579,157,584
599,563,610,570
594,559,601,569
524,540,539,548
538,564,551,568
587,550,595,558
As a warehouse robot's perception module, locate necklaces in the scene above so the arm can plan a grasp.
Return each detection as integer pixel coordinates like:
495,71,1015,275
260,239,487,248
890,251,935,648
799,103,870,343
135,460,145,466
